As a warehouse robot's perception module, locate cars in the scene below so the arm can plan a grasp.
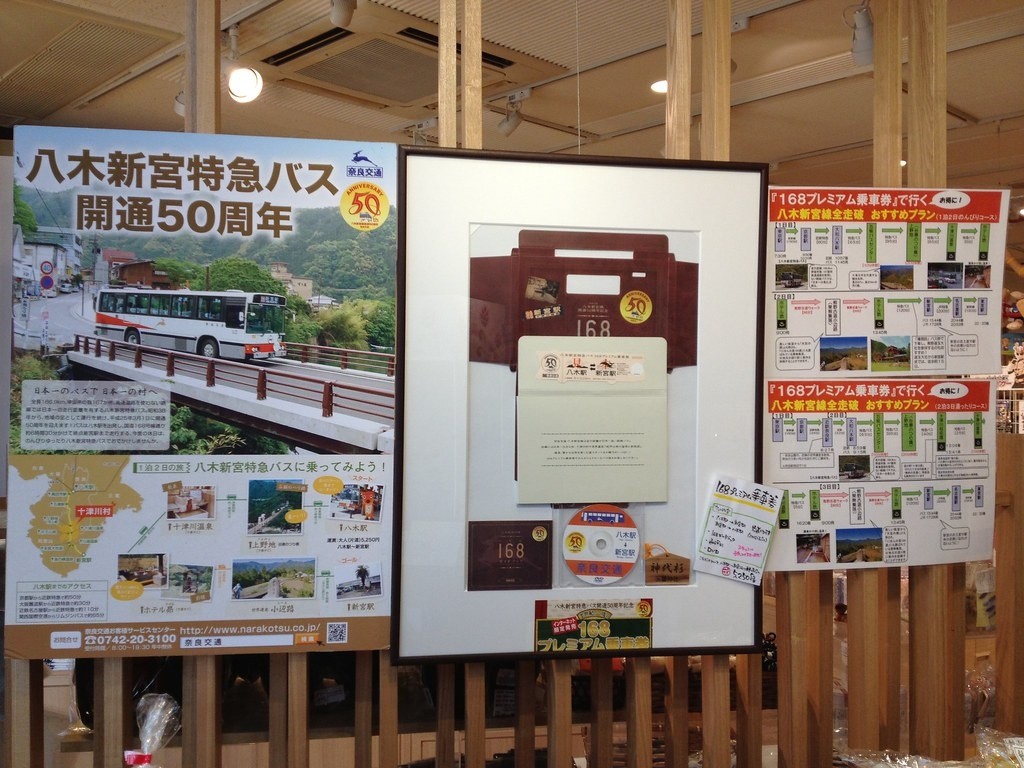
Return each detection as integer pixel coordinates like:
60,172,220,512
42,283,84,298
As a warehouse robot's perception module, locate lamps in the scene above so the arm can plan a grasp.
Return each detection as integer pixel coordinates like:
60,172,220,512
221,23,264,104
842,0,876,67
328,0,359,29
495,88,533,137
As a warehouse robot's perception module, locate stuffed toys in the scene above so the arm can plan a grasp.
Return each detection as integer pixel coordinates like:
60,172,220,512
1004,288,1024,330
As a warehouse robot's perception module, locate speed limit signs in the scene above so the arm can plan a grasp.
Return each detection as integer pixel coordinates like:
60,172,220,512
40,261,53,275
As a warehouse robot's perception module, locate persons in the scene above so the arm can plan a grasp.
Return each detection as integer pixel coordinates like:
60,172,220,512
233,582,243,598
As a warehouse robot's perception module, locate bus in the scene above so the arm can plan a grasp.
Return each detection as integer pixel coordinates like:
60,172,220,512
338,484,382,513
781,272,803,287
95,287,287,363
944,274,956,283
